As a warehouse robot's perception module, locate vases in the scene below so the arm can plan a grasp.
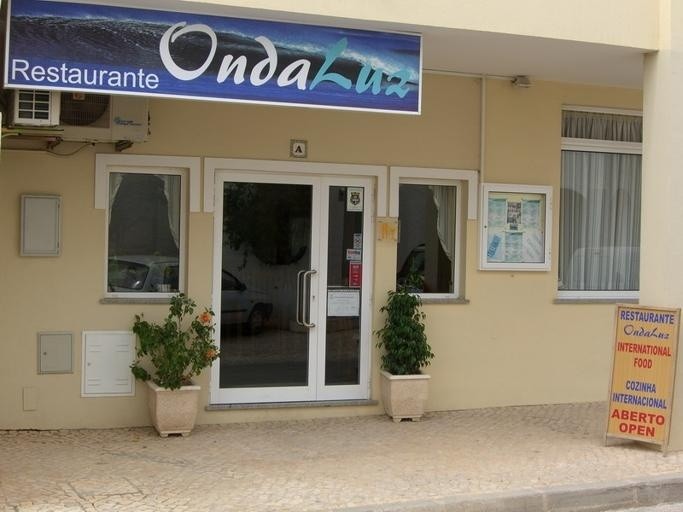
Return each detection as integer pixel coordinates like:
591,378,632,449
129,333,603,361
147,381,202,437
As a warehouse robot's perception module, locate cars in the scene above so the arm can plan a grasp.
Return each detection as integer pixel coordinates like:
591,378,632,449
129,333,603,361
108,254,273,335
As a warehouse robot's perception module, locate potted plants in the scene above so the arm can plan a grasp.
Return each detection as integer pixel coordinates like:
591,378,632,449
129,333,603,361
372,281,435,422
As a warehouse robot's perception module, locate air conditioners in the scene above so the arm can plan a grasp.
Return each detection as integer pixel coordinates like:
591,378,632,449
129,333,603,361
8,86,150,144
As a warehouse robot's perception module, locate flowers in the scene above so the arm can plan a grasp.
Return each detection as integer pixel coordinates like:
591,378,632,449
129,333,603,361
129,293,225,390
